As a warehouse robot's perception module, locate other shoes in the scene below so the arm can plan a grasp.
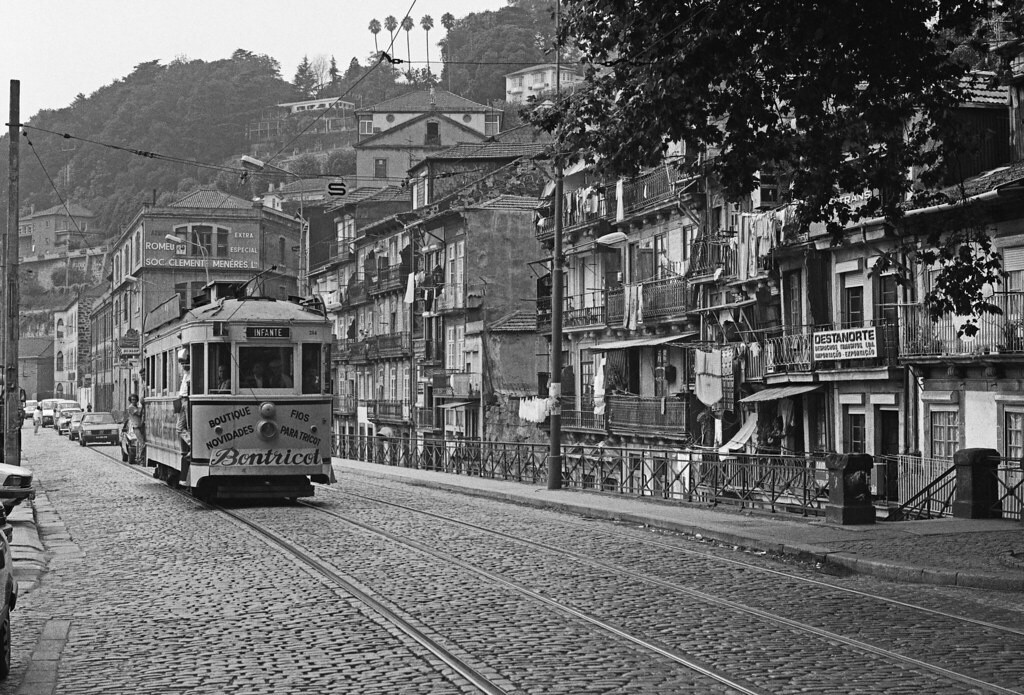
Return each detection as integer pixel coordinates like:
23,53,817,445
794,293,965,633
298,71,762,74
35,433,40,435
137,457,144,463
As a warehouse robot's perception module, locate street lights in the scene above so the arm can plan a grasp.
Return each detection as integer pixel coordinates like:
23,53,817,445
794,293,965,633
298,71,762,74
164,233,209,286
547,230,630,490
125,275,175,295
240,154,309,303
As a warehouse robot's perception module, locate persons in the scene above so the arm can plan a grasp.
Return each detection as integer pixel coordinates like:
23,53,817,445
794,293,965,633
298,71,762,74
139,368,145,434
176,348,191,459
248,364,269,389
33,406,41,435
269,359,291,388
218,365,231,395
128,394,145,462
87,403,92,412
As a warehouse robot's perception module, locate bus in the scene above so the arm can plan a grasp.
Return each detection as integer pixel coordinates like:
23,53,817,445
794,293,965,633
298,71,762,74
141,292,338,500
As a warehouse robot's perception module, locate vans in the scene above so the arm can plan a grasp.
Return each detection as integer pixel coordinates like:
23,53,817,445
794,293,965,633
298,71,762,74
23,400,37,420
52,403,83,427
38,399,67,428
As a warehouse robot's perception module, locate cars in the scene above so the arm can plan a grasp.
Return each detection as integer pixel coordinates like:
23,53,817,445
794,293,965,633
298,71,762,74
120,419,157,466
57,408,84,435
78,413,119,447
67,412,85,441
0,462,37,518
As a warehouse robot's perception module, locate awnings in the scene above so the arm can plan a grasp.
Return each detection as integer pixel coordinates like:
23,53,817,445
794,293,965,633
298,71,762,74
737,387,817,401
585,330,700,349
